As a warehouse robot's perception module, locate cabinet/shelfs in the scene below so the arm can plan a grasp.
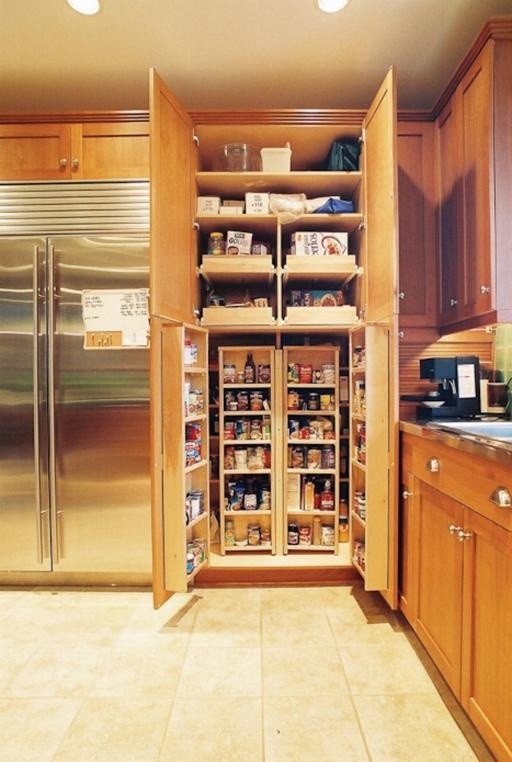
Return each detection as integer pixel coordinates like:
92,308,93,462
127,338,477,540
406,436,512,761
435,13,511,336
148,67,400,330
148,317,399,611
0,110,151,181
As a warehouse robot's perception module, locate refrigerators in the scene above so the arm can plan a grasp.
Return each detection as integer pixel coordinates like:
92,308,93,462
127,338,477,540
1,234,151,571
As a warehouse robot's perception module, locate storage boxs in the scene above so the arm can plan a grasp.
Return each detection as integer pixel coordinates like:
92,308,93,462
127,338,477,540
226,231,253,255
260,148,292,173
197,192,269,216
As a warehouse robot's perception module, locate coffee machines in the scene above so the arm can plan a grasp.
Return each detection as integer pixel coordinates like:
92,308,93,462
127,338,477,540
416,355,480,419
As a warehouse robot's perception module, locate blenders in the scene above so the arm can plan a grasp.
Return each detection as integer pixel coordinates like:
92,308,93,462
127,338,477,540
479,360,508,416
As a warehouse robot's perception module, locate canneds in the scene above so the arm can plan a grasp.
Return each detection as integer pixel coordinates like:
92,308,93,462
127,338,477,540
207,231,228,257
308,392,320,410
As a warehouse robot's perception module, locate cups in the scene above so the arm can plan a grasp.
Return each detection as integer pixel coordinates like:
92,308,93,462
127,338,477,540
234,446,247,469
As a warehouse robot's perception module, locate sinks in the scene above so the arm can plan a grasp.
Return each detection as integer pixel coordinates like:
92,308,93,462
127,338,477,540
436,420,512,440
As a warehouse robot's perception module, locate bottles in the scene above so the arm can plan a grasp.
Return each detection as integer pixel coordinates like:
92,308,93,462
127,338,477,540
321,444,335,469
230,391,263,411
208,231,225,255
224,352,271,383
258,483,270,510
320,479,334,511
304,477,314,510
293,447,305,469
312,364,335,384
294,364,300,383
184,338,193,367
314,494,320,510
312,516,322,546
243,479,258,510
